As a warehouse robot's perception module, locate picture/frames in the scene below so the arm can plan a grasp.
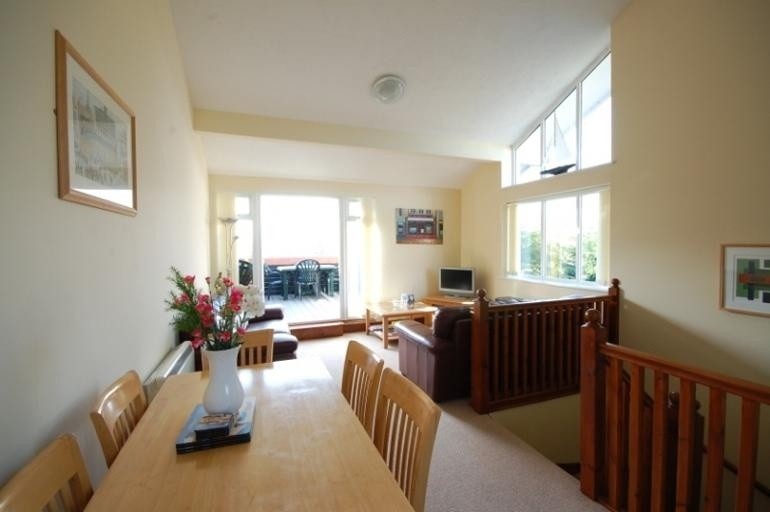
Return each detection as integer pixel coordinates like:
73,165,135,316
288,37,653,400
718,243,770,318
54,29,140,217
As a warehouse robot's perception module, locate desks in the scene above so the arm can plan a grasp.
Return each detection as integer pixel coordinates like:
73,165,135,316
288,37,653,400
422,294,478,327
276,264,337,300
364,301,438,349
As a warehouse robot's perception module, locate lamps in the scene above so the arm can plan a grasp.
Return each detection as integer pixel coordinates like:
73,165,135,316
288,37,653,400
372,75,407,106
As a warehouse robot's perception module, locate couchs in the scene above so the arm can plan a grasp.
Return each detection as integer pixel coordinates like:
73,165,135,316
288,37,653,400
393,293,583,404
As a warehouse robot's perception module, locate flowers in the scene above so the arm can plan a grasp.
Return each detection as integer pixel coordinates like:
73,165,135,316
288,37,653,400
164,264,266,351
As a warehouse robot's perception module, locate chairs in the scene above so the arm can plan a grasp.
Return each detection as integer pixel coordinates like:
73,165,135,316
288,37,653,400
179,304,298,371
293,259,320,301
263,262,284,300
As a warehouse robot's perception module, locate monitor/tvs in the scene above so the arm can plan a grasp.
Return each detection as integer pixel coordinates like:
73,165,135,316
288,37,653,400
439,266,476,300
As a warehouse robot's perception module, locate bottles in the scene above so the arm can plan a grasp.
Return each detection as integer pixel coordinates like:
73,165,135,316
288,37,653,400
393,293,414,311
395,208,443,245
